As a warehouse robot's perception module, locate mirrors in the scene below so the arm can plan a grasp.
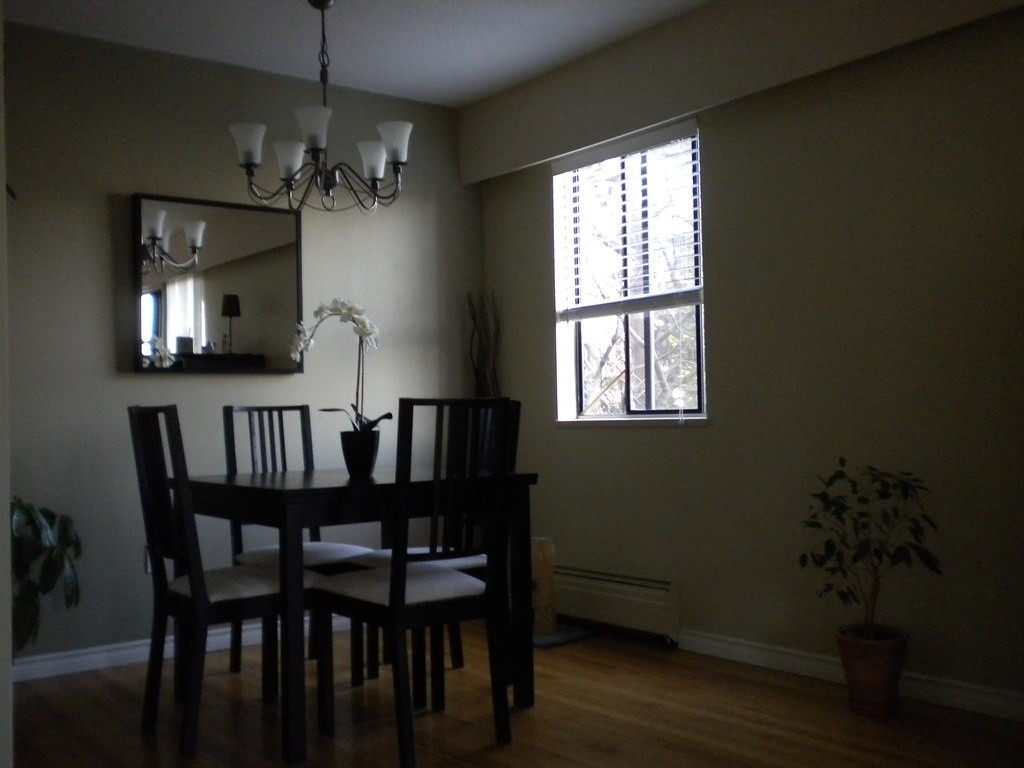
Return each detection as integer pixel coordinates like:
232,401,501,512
133,192,304,373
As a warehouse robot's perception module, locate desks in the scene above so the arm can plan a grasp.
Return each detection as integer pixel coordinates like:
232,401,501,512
165,470,539,768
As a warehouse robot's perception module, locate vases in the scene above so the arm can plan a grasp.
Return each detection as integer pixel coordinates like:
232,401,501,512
339,431,380,478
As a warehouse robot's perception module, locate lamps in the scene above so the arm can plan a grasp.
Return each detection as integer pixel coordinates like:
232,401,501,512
140,200,206,275
221,293,242,354
229,0,412,215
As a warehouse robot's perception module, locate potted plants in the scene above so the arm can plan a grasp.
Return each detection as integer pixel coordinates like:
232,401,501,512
800,457,945,716
10,496,82,667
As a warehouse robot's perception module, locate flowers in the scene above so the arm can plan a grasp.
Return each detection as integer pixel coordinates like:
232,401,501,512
148,333,177,369
290,300,393,430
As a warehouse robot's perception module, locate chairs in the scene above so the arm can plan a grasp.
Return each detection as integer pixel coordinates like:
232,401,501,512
304,398,524,768
127,404,375,768
